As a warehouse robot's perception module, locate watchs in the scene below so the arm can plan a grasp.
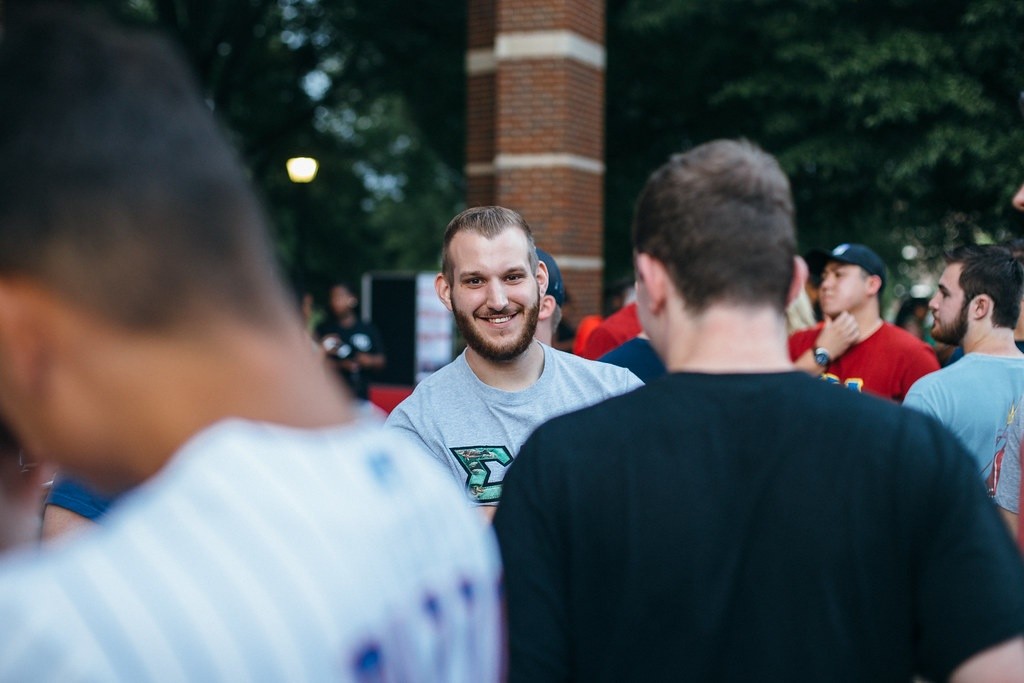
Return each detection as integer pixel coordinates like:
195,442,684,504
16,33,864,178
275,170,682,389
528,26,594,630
811,343,832,368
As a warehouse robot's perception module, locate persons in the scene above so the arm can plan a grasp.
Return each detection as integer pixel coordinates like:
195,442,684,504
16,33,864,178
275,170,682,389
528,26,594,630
0,0,503,683
383,139,1024,683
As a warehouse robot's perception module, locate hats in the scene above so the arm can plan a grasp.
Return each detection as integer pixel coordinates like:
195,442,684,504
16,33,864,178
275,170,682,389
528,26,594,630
533,246,563,302
803,243,891,287
